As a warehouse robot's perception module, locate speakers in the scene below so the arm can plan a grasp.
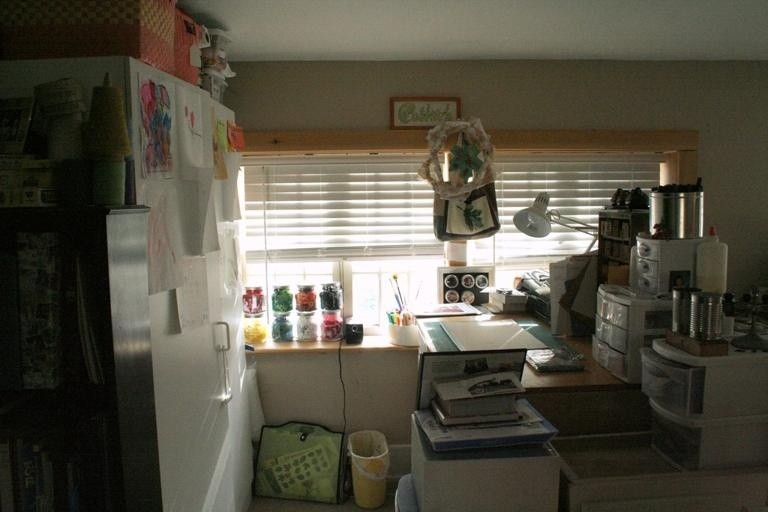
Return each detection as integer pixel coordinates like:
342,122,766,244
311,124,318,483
344,323,363,345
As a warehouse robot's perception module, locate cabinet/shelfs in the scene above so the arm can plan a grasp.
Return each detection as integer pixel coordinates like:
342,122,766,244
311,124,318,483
638,339,707,470
1,202,165,510
634,235,661,292
0,59,259,512
547,431,767,509
591,286,630,384
596,209,634,267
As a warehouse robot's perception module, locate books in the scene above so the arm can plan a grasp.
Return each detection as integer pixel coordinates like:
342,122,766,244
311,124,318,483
600,219,630,262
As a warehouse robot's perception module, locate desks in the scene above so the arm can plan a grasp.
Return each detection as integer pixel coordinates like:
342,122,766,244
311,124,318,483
417,299,652,430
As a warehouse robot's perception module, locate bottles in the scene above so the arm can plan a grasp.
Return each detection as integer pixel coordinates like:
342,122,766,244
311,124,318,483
319,283,341,310
270,285,294,313
296,312,317,342
242,286,265,314
321,311,344,341
295,284,316,311
272,312,294,342
686,288,737,340
244,313,266,345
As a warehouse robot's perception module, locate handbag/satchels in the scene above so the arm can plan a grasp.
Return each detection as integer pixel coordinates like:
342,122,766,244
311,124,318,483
251,422,352,504
433,181,501,240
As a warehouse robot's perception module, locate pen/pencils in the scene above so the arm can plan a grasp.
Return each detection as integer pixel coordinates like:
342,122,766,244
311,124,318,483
386,311,409,325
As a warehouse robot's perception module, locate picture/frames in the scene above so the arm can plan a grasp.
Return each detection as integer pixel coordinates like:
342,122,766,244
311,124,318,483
388,94,461,132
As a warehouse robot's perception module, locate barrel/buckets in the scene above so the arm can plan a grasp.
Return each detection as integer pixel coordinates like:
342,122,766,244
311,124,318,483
690,292,723,341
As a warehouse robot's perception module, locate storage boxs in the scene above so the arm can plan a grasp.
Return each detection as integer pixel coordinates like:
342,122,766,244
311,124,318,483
411,413,561,509
201,27,233,71
204,71,231,107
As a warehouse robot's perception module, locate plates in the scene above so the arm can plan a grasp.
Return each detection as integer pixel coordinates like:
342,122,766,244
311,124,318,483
444,274,489,304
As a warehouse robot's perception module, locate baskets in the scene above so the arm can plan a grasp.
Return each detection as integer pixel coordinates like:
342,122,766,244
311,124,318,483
175,9,202,85
1,0,174,76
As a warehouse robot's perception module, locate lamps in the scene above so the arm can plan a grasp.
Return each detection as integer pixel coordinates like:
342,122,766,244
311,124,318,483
512,191,600,256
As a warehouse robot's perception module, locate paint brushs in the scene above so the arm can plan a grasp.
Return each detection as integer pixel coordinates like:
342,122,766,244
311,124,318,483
389,275,405,312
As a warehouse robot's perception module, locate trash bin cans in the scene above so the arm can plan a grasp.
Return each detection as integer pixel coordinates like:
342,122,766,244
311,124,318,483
348,430,389,509
